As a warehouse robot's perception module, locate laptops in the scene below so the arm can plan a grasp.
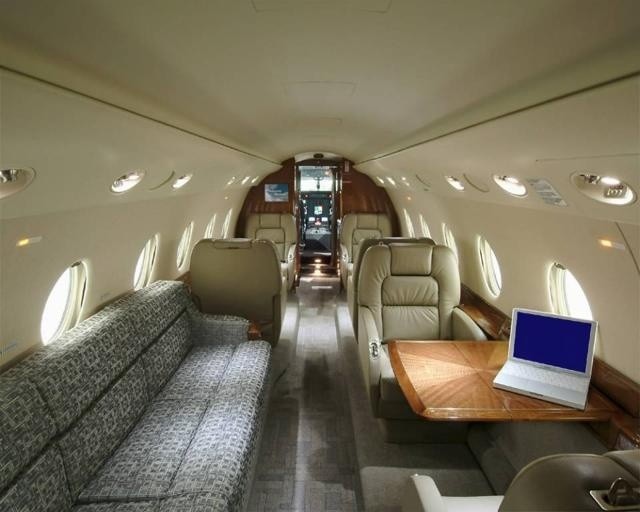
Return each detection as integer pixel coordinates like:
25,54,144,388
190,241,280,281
492,307,599,412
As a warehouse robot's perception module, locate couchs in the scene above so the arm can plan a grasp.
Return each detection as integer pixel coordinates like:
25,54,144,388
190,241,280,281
0,278,273,512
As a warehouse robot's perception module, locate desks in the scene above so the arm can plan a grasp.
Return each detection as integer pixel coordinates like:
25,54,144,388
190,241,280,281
388,337,624,423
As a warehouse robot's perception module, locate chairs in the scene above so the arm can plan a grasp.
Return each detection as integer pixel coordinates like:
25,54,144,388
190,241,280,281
340,210,490,447
189,238,288,346
245,213,298,294
